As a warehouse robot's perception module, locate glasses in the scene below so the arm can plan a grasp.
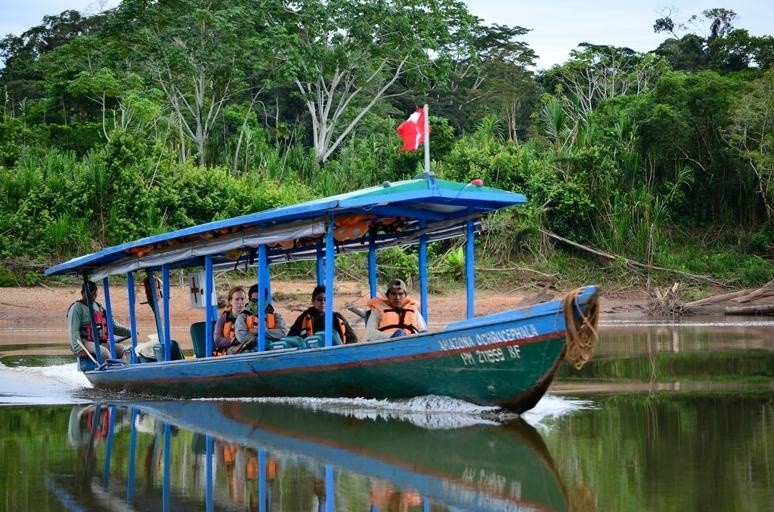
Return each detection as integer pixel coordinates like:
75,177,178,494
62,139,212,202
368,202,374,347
250,298,257,303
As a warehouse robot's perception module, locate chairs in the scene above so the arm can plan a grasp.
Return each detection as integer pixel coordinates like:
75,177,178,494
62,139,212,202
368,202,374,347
153,339,184,362
190,321,217,358
266,329,342,350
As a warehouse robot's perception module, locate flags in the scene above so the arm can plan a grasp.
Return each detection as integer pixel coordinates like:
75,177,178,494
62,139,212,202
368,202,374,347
395,107,426,152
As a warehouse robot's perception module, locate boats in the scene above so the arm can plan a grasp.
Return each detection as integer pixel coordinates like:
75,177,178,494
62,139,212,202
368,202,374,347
41,173,604,417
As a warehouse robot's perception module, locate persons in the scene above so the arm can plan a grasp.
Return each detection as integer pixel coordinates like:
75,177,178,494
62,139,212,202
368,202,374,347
213,439,278,512
214,287,246,355
287,286,357,344
305,460,341,499
234,283,288,348
368,480,421,512
66,280,132,364
67,402,106,473
144,270,163,319
344,301,366,318
364,277,428,341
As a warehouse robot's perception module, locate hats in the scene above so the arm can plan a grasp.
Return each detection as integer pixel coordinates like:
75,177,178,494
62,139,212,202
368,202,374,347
385,279,408,296
